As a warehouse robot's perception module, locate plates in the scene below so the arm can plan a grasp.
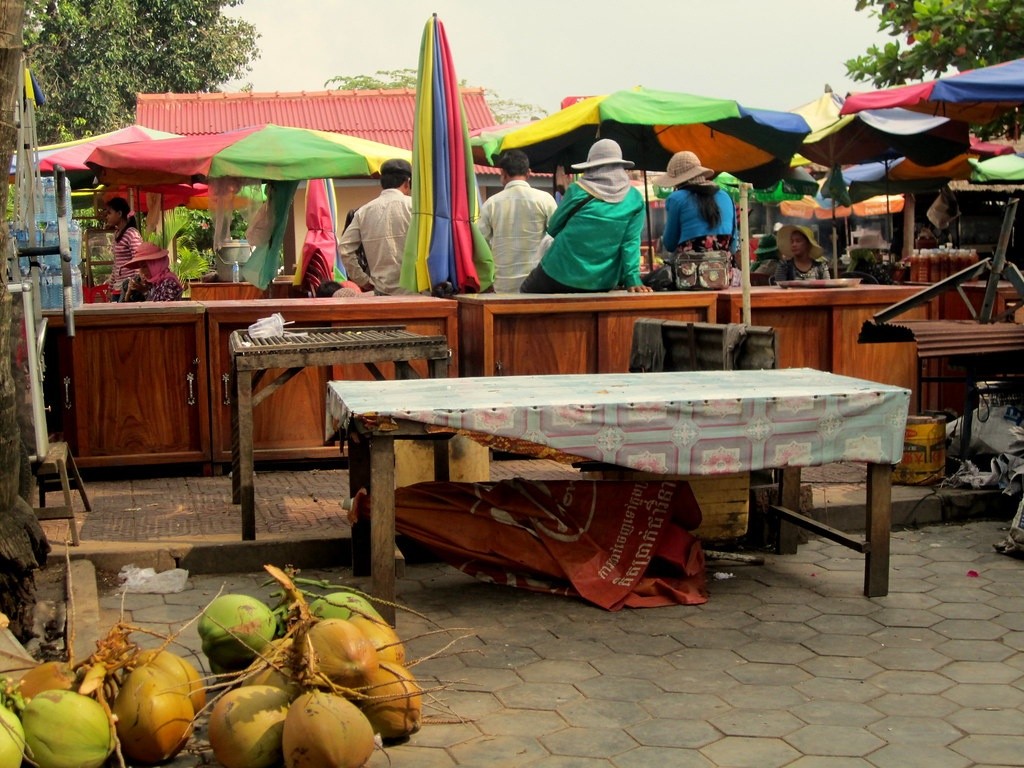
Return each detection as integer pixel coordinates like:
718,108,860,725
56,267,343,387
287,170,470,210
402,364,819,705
775,278,863,287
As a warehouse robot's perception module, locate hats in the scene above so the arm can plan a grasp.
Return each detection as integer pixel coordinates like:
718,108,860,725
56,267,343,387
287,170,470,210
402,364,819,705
845,229,891,252
124,241,169,270
753,233,778,254
776,225,823,259
571,139,634,169
651,151,714,187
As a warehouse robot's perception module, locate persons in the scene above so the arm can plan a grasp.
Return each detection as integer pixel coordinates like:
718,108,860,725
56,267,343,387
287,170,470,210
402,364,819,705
775,224,830,284
642,151,738,290
750,234,784,276
519,139,655,294
315,208,374,298
339,159,422,296
106,198,143,300
120,242,182,301
844,230,893,285
478,148,559,294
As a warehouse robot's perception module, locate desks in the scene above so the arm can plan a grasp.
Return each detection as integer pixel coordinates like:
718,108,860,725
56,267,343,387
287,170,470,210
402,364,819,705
323,366,910,629
228,330,450,539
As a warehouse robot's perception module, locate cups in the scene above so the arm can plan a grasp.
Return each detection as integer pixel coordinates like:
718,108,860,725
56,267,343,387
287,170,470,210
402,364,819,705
248,313,285,338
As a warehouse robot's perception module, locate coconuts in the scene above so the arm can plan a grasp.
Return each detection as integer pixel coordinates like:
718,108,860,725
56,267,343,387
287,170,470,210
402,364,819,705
0,591,423,768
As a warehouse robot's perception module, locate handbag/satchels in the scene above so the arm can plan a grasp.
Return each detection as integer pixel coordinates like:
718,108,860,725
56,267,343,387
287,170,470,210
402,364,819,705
672,250,732,289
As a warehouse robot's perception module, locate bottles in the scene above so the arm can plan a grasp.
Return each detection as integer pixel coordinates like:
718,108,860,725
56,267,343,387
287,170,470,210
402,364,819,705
910,243,980,285
232,260,240,283
5,175,87,310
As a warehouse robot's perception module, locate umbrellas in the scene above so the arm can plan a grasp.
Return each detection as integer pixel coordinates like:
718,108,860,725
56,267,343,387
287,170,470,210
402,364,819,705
399,13,496,297
470,59,1024,279
8,123,414,244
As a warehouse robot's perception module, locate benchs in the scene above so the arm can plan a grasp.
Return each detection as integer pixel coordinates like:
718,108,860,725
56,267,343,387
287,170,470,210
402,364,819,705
28,442,91,546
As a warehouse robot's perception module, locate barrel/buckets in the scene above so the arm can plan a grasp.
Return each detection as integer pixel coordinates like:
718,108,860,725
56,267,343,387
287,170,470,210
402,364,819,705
891,414,947,485
976,380,1024,428
215,239,254,282
